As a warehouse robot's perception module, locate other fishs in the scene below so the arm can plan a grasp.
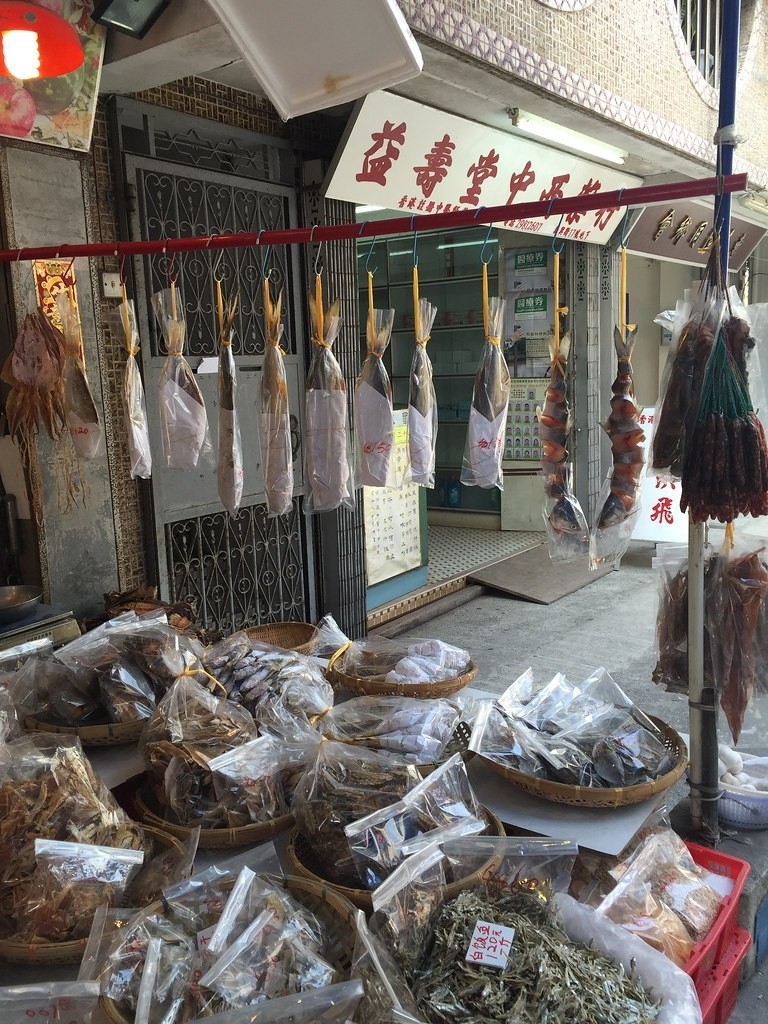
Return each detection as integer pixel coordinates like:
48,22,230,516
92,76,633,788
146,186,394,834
595,324,646,531
307,295,347,507
216,292,245,514
355,310,389,486
470,307,507,483
124,328,150,475
540,311,586,536
211,644,286,721
408,307,433,480
158,317,204,468
259,285,290,513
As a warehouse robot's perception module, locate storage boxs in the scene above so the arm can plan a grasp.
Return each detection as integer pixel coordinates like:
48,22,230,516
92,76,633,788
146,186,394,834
433,349,479,373
681,840,751,997
437,399,471,419
432,310,485,327
488,488,500,511
698,927,751,1024
430,478,460,509
403,313,415,328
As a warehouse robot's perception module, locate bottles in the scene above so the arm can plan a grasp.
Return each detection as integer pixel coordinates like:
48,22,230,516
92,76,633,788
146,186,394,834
438,478,446,506
448,474,463,508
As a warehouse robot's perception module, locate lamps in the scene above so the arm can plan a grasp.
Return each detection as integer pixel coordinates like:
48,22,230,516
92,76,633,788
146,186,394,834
737,191,768,216
506,106,629,165
89,0,173,42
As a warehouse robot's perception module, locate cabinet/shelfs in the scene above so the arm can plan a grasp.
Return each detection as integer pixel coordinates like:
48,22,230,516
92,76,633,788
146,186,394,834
357,225,500,531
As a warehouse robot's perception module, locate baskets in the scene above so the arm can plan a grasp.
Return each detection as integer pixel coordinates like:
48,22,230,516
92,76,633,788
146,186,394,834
0,598,768,1024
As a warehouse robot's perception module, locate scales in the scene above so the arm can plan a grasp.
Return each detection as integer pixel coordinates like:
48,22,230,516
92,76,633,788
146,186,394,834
0,603,82,663
